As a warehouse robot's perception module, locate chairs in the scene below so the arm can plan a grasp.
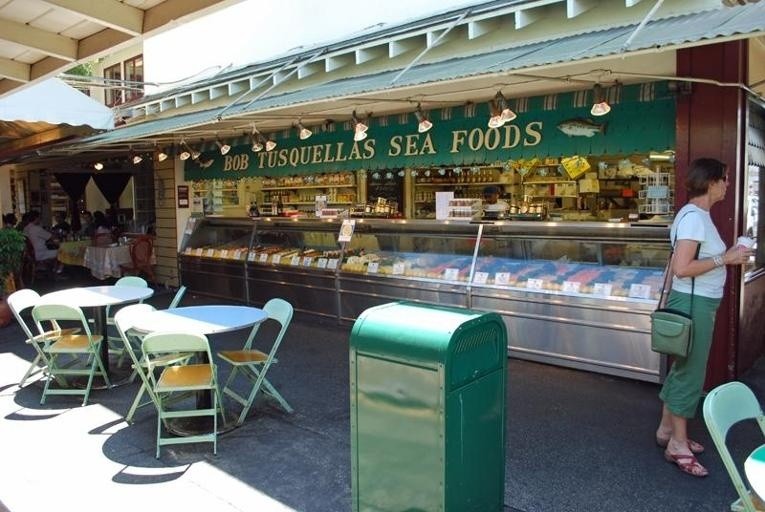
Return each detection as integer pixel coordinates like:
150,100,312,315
698,380,764,511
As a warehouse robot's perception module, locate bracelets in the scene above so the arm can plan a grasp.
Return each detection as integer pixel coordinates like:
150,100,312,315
711,251,725,268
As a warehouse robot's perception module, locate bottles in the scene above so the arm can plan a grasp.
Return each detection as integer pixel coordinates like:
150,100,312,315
454,186,484,199
449,200,477,218
415,190,435,200
509,195,542,214
49,228,81,240
350,201,398,218
264,173,353,186
416,169,493,182
299,190,315,203
249,201,258,216
264,190,290,203
259,204,283,215
303,207,315,216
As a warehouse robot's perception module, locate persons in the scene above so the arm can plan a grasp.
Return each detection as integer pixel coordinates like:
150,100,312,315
481,186,508,209
0,206,138,291
650,159,752,477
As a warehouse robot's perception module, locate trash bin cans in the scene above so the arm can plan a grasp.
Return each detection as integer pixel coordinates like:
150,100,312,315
346,302,507,512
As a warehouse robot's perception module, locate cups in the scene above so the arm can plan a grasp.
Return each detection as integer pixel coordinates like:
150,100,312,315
736,236,755,249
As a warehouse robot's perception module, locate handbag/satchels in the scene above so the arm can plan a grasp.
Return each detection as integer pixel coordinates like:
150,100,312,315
650,310,694,359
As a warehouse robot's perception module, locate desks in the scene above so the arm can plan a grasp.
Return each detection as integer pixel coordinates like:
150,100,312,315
742,439,764,503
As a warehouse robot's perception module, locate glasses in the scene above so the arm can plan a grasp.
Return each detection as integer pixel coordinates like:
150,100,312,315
720,175,727,182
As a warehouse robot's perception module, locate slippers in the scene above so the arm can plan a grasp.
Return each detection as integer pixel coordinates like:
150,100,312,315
655,437,705,456
665,449,708,476
